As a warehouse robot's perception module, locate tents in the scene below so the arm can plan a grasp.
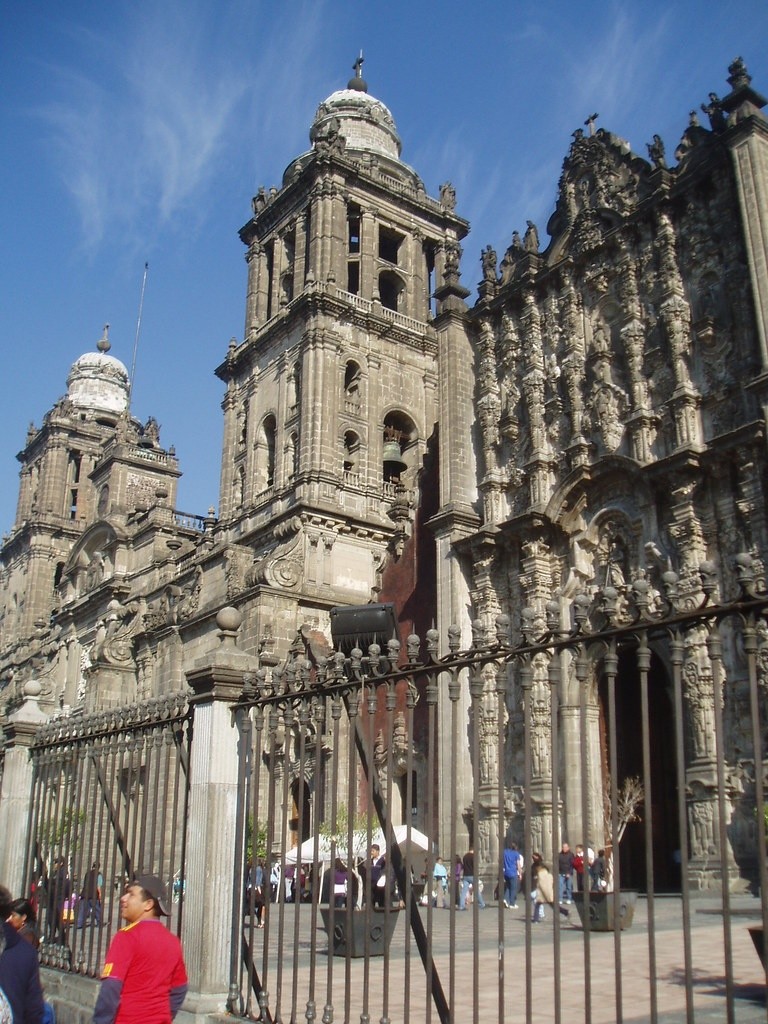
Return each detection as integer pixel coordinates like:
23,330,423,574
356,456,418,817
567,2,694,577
275,825,436,904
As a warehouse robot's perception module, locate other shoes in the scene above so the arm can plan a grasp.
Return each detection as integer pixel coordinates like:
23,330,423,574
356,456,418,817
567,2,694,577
461,907,468,911
97,922,108,928
559,901,563,905
566,899,571,904
481,903,489,909
509,905,519,909
503,899,508,908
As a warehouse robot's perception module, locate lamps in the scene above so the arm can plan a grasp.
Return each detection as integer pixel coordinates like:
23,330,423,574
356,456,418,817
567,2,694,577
326,605,403,677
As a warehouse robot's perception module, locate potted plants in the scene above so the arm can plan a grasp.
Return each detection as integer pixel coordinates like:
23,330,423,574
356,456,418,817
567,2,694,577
747,800,768,978
318,800,406,960
574,776,643,931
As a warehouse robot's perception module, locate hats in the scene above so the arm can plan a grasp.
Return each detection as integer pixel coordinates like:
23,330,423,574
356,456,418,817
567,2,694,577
53,856,66,864
128,874,172,916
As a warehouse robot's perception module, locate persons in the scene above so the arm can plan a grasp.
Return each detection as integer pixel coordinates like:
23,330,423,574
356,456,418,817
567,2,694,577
244,839,611,930
0,847,109,1024
92,874,189,1024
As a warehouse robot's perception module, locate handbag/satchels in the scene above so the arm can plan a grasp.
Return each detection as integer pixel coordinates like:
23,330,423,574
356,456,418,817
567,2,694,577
334,884,345,894
270,868,278,884
